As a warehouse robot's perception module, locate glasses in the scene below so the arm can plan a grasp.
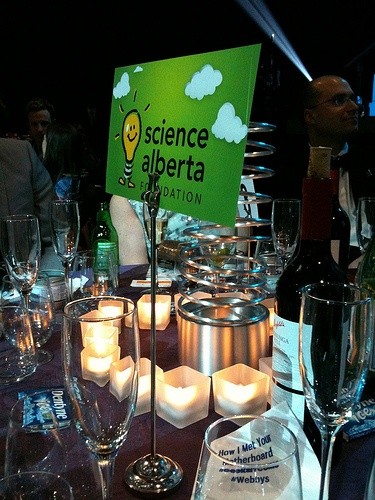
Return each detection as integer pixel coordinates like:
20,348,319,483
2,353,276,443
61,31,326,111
309,94,357,109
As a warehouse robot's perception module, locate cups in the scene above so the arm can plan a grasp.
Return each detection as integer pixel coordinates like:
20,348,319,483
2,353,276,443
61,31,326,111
0,273,57,345
356,198,375,253
0,306,37,385
64,250,96,301
190,414,303,500
0,388,74,500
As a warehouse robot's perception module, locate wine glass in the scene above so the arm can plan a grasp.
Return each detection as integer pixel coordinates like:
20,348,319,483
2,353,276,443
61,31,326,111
298,283,375,499
0,214,53,367
252,200,302,298
63,295,141,500
51,200,80,308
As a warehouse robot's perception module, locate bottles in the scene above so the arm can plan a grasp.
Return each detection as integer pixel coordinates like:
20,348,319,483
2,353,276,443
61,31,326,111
93,210,119,278
272,146,351,395
348,233,374,375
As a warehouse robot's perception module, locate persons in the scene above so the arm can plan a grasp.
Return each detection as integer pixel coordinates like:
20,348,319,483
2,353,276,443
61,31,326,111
270,76,375,261
0,100,234,279
